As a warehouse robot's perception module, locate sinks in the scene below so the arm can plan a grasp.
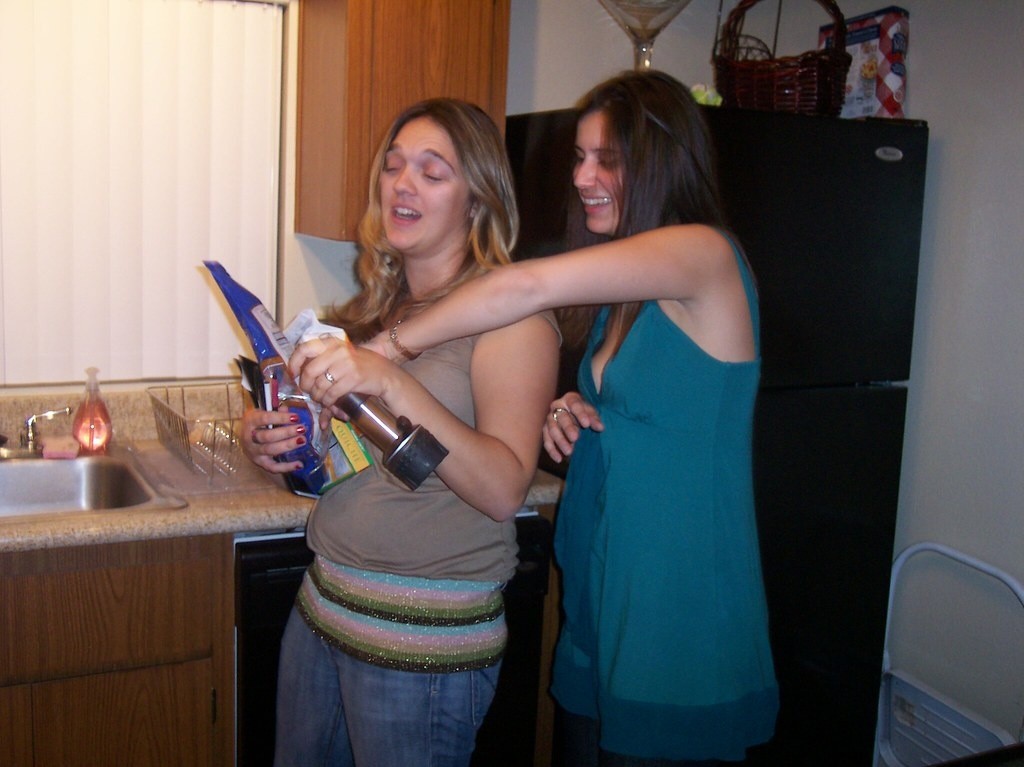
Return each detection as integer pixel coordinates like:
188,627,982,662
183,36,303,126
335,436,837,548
0,457,152,516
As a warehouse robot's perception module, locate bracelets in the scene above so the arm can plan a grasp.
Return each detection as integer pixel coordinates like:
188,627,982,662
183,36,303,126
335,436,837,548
389,320,418,360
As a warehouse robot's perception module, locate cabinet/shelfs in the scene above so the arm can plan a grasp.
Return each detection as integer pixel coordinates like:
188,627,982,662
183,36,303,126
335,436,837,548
0,534,230,767
294,0,512,242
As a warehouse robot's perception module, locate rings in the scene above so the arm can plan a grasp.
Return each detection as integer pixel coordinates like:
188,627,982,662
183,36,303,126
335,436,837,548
325,370,338,385
553,408,567,422
252,429,259,442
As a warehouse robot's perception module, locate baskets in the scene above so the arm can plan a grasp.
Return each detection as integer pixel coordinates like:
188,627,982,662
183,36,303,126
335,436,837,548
147,380,245,486
711,0,853,118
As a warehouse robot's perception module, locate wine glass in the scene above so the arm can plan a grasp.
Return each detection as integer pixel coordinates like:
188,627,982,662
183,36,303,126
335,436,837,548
598,0,695,70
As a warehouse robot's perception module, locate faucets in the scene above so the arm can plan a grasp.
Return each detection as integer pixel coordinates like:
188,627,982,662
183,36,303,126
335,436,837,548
19,406,72,453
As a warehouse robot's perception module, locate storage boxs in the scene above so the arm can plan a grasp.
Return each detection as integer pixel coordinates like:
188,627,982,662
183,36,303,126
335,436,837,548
818,6,908,118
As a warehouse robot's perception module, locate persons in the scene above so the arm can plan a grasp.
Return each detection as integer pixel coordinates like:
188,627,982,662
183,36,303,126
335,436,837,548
240,98,562,767
321,70,778,767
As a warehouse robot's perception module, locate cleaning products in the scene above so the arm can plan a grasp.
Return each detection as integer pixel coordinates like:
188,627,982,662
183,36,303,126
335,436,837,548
72,366,112,454
